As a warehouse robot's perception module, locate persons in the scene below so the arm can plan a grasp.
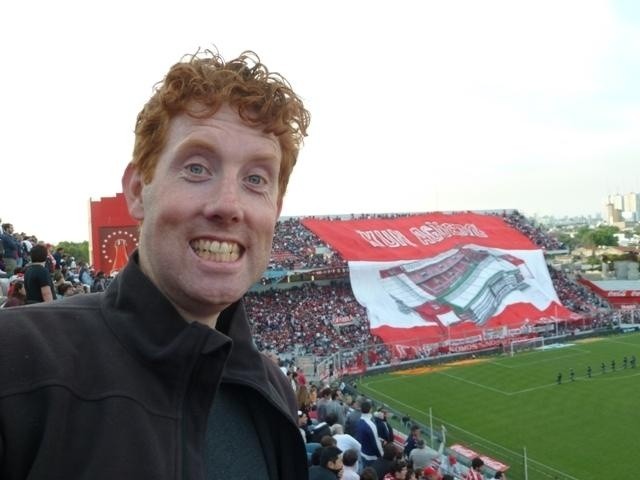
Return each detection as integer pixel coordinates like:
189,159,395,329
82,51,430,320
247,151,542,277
0,49,309,480
243,209,640,480
0,223,114,307
